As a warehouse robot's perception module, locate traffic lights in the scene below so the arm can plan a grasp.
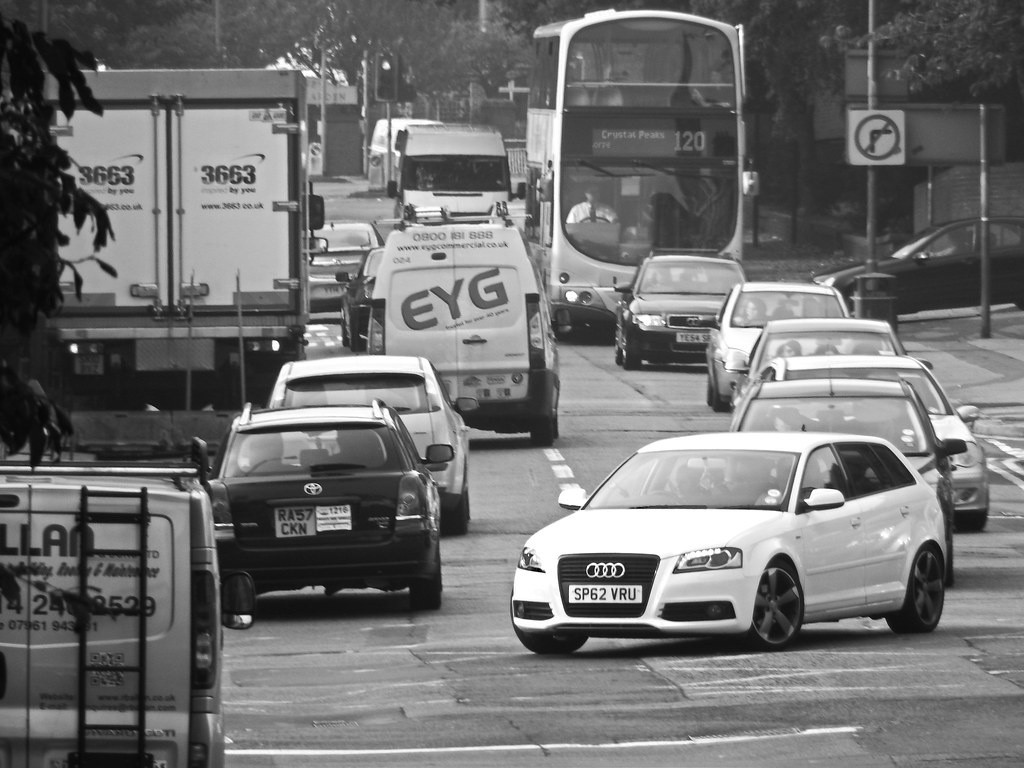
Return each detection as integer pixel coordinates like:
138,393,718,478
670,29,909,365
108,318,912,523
377,49,399,103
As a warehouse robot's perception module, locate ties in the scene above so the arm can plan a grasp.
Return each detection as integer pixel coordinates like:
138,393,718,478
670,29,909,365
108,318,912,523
589,204,597,223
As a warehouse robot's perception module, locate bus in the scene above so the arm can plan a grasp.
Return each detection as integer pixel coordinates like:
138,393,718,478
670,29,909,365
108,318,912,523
525,7,759,335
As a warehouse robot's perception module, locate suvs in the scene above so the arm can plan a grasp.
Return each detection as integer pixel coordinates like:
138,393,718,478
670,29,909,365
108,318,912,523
614,248,746,371
0,435,258,768
264,355,481,534
208,398,455,610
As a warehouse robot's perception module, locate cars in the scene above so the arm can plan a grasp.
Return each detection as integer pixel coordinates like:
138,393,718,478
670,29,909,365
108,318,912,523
804,217,1024,318
510,431,948,654
706,280,989,532
303,218,386,352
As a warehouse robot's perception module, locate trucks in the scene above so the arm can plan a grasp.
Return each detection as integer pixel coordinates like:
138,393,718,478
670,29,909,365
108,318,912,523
366,200,561,446
367,116,528,232
28,67,327,373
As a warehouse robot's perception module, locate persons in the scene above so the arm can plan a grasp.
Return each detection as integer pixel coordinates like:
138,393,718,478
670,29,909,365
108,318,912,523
925,227,969,257
648,265,676,291
740,298,766,326
756,460,808,509
780,341,798,357
566,185,619,224
